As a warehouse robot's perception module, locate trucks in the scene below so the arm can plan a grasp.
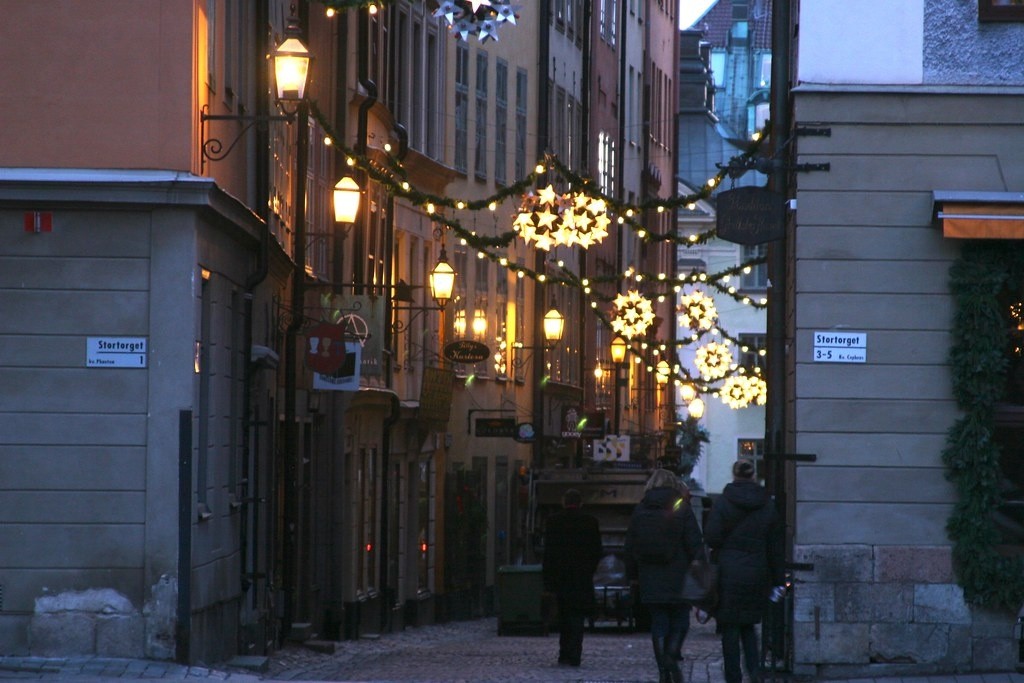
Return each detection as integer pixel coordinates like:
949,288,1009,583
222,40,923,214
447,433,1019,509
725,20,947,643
536,467,703,632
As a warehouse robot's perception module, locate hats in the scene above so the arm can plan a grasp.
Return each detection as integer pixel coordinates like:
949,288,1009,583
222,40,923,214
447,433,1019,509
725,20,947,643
733,459,754,481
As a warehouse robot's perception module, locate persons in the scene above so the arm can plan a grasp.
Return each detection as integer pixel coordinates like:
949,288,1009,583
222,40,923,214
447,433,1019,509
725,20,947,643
541,489,603,667
702,458,785,683
624,468,707,683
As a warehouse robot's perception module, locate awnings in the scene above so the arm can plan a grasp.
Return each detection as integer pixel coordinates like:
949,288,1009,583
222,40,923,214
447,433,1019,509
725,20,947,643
931,190,1024,239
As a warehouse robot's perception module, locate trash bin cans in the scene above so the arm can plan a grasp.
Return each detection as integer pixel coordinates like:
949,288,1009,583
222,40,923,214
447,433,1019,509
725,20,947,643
496,564,551,636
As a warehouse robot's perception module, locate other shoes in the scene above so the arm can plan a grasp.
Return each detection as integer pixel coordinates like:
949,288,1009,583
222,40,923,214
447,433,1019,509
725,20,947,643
558,651,581,666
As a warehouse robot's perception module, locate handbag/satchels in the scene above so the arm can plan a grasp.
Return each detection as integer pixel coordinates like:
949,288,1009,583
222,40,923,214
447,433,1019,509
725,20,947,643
686,543,720,622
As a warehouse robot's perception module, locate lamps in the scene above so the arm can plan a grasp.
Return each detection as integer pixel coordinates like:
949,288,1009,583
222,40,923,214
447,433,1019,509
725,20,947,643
584,337,627,386
389,243,456,336
302,171,360,252
511,305,565,377
200,18,317,175
631,357,705,432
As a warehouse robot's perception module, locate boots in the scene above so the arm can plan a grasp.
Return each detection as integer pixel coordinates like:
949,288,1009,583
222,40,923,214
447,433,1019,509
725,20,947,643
652,636,672,683
665,631,686,683
